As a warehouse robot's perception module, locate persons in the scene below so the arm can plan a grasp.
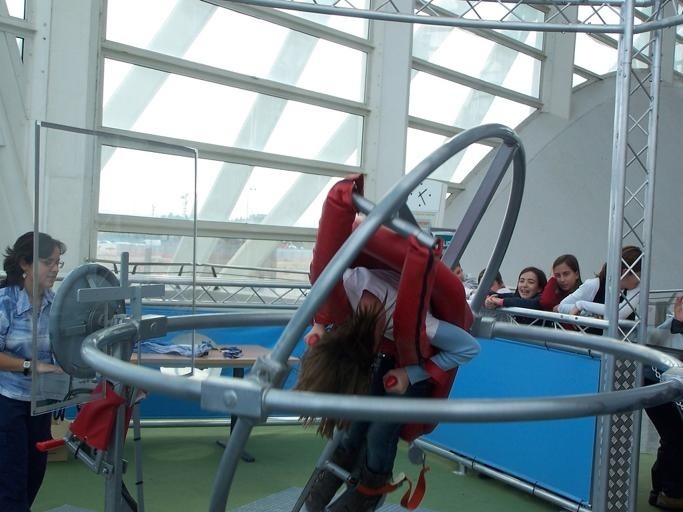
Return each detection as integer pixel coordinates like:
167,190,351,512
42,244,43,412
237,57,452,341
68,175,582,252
641,295,683,511
484,267,552,328
466,268,512,297
558,244,643,336
450,263,475,299
304,263,478,512
539,254,583,330
0,231,68,511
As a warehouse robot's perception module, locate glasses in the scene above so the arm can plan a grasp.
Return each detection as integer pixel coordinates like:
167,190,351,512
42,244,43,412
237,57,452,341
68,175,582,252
40,258,64,268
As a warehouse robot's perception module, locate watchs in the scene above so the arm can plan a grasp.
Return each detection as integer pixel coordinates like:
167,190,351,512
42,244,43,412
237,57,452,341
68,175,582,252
23,359,32,375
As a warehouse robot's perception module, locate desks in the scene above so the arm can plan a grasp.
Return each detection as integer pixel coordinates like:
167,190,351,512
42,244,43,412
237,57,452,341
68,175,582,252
73,341,295,464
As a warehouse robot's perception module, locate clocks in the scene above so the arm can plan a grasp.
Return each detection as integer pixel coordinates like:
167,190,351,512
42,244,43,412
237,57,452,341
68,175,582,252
404,178,443,212
